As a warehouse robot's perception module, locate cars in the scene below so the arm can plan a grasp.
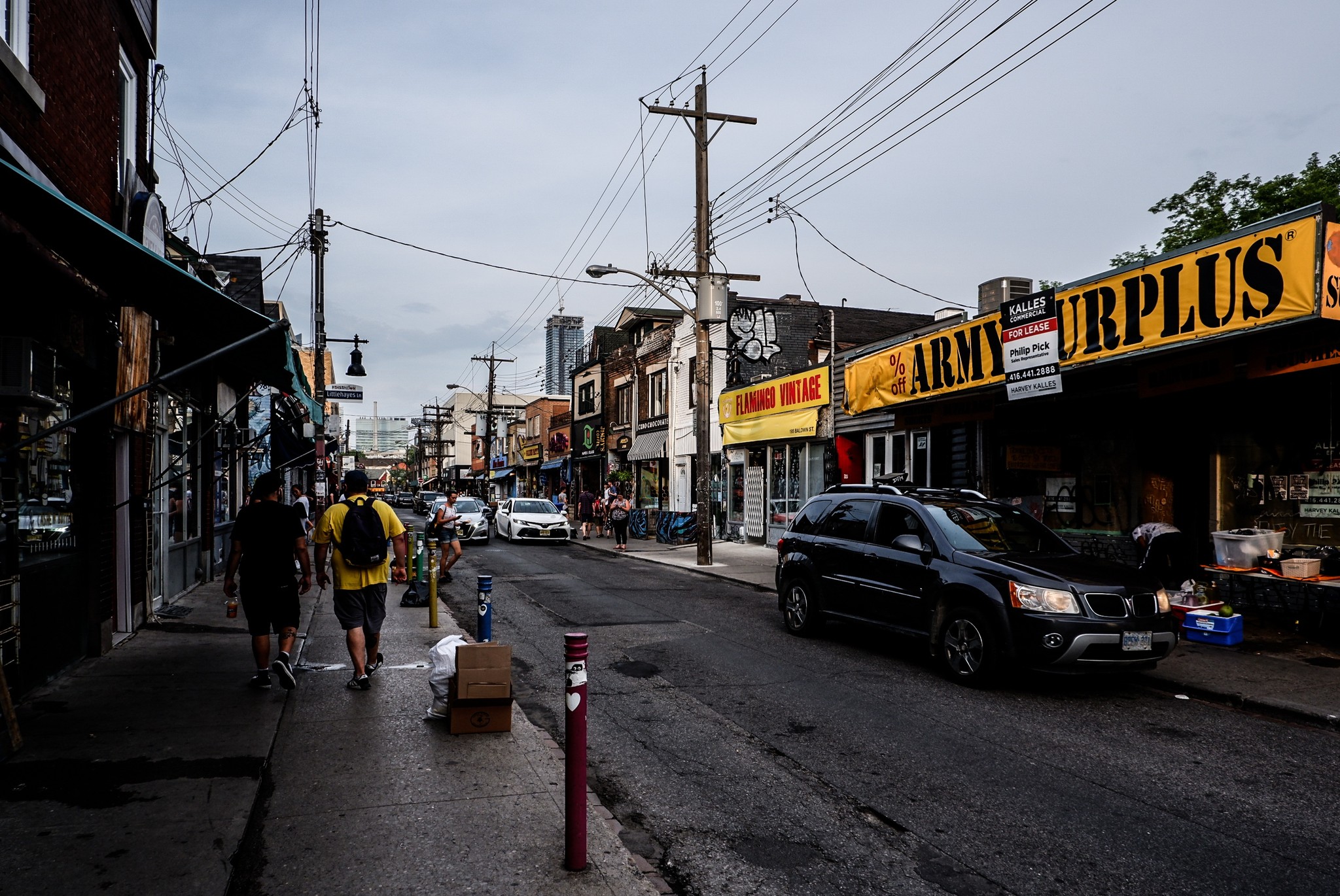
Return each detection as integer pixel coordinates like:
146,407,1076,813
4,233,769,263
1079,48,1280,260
422,496,490,546
494,497,571,546
373,490,445,516
464,497,493,524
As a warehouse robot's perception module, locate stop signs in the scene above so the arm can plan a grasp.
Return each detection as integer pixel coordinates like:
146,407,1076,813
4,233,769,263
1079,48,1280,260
417,478,423,484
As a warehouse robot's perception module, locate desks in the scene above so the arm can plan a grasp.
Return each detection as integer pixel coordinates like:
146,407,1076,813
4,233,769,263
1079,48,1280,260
1204,563,1340,630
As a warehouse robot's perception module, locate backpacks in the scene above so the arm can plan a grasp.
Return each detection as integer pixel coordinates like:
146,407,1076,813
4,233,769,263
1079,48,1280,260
329,497,391,569
595,498,603,513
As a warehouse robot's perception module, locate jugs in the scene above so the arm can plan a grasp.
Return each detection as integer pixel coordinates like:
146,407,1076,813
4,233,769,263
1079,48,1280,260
1195,586,1207,606
1181,591,1199,607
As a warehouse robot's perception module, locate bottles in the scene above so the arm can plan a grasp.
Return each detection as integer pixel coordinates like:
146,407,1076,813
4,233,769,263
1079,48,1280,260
225,591,238,621
1253,526,1260,536
1228,529,1233,534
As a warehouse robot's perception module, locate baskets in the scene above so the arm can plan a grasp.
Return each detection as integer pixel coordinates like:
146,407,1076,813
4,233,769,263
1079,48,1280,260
1280,558,1321,578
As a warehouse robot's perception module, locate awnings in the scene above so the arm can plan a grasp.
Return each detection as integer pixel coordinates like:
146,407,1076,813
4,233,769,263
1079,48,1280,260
492,469,515,478
0,157,329,509
409,477,436,488
627,429,669,461
540,455,568,470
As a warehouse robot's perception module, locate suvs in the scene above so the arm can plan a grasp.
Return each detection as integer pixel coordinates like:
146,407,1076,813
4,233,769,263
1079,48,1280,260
774,471,1178,691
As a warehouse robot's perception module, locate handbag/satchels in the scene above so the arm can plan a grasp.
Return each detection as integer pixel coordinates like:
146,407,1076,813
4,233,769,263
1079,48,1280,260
609,487,617,503
1289,545,1338,571
427,504,447,538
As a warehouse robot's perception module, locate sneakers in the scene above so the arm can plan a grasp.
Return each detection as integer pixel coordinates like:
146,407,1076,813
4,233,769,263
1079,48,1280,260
271,651,296,690
347,671,371,690
250,670,272,688
365,653,383,677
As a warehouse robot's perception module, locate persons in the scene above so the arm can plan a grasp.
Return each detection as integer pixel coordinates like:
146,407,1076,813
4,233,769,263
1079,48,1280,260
629,493,632,509
219,486,251,520
1130,521,1185,593
577,480,618,540
436,490,462,582
558,486,575,530
223,469,407,690
610,491,630,549
169,481,198,543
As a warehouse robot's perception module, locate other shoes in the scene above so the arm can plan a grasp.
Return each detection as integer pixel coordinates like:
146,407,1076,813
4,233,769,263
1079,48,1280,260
583,536,586,540
444,571,453,580
295,559,301,569
620,547,626,549
580,527,582,529
613,546,621,549
596,535,600,538
587,536,590,539
607,534,609,538
438,577,452,583
610,536,613,538
600,534,604,537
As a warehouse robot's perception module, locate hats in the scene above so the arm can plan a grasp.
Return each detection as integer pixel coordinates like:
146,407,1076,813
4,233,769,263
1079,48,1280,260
255,472,286,495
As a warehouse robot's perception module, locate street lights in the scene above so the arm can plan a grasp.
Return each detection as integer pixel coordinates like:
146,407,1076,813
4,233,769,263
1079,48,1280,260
395,438,423,490
314,334,369,521
446,383,492,503
585,263,713,566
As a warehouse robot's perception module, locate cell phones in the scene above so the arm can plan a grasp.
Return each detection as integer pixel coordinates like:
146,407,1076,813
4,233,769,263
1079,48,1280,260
615,505,618,506
457,516,462,517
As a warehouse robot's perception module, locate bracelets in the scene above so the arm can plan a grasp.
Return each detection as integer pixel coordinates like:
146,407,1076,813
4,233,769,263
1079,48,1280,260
396,567,405,569
578,511,580,513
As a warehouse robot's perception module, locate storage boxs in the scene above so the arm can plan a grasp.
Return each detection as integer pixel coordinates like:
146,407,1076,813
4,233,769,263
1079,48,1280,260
1280,558,1321,578
1170,598,1225,634
1257,554,1295,570
1182,609,1244,646
1211,528,1285,569
448,640,515,735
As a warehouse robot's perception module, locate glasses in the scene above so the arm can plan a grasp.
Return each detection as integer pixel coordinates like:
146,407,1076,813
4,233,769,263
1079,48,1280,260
280,487,285,490
